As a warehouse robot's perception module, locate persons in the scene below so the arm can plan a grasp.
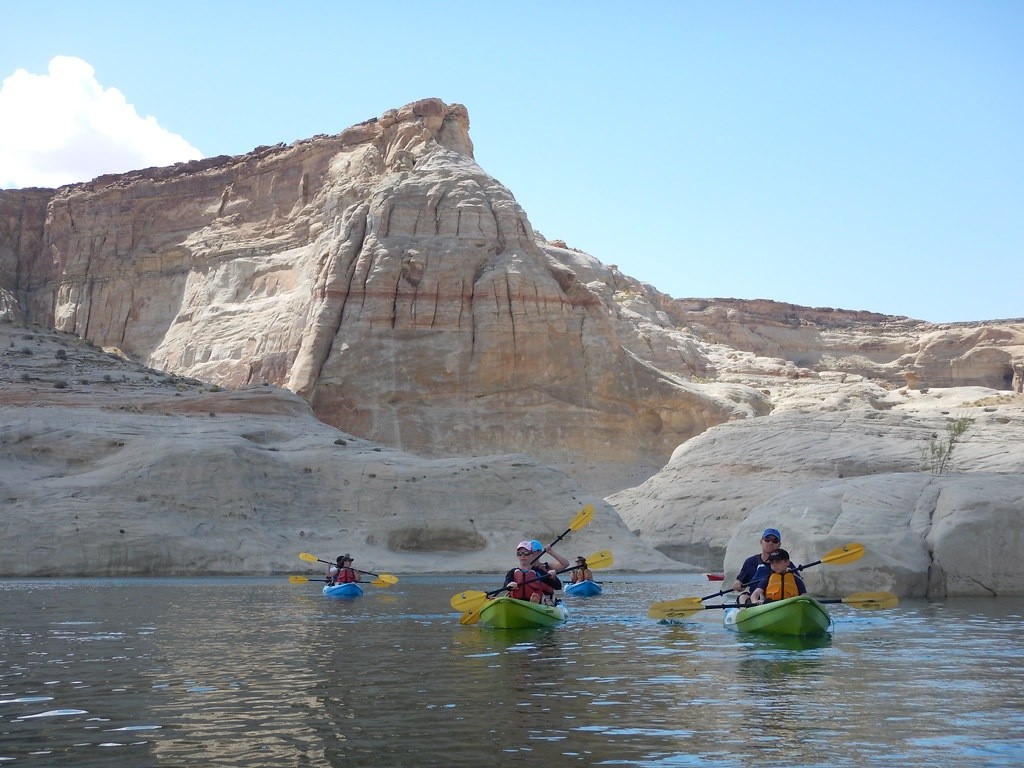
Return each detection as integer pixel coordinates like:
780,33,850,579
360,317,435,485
325,554,362,585
487,539,570,604
733,528,808,609
570,556,592,583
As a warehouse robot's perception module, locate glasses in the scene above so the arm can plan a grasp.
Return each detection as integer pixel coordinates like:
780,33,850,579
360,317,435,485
763,537,780,543
517,551,531,556
575,561,580,562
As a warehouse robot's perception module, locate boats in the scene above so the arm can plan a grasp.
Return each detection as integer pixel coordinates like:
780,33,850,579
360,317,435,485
479,597,568,628
563,580,603,596
724,595,832,636
707,573,724,580
323,583,363,597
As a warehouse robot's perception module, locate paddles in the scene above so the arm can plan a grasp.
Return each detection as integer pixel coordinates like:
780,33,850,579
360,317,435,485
449,550,614,612
459,504,595,625
298,552,399,584
288,575,390,588
681,542,865,604
647,590,900,620
560,580,633,585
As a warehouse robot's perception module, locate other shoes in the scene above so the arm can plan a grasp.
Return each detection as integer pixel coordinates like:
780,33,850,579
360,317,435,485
745,598,752,608
762,598,773,604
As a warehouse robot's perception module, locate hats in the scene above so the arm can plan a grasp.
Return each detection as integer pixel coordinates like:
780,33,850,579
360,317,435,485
762,528,780,539
516,541,532,552
529,540,543,552
766,549,789,561
341,554,354,562
